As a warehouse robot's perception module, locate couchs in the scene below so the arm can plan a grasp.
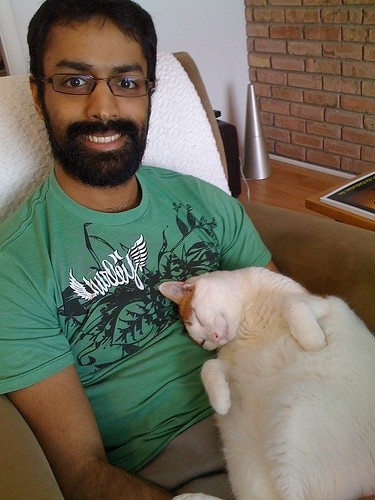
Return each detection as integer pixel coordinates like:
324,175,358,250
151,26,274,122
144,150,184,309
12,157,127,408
0,51,375,500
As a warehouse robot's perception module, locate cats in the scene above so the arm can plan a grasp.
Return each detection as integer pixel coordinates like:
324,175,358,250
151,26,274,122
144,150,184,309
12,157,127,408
157,264,375,500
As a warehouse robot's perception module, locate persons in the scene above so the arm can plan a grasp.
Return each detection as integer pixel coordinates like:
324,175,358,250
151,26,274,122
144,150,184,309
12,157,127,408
1,1,279,500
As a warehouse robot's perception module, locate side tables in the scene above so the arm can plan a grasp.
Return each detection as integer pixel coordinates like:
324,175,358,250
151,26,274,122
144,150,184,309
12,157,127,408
305,168,375,231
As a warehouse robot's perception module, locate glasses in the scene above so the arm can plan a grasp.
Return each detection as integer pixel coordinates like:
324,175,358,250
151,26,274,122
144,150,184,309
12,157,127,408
35,73,155,97
